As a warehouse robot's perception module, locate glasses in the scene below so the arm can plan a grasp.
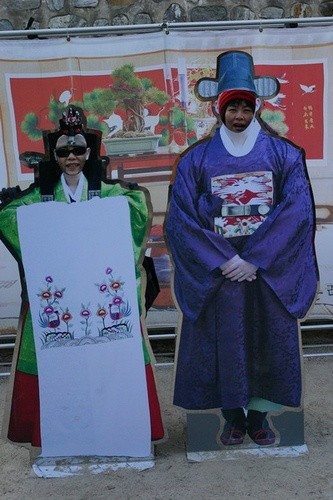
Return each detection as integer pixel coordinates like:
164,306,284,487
55,145,88,158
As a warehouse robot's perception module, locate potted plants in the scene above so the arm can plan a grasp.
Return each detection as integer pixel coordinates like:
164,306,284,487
83,62,172,157
186,68,219,141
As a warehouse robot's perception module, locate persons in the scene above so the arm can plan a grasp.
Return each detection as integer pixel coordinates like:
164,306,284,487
219,88,256,133
53,134,91,176
